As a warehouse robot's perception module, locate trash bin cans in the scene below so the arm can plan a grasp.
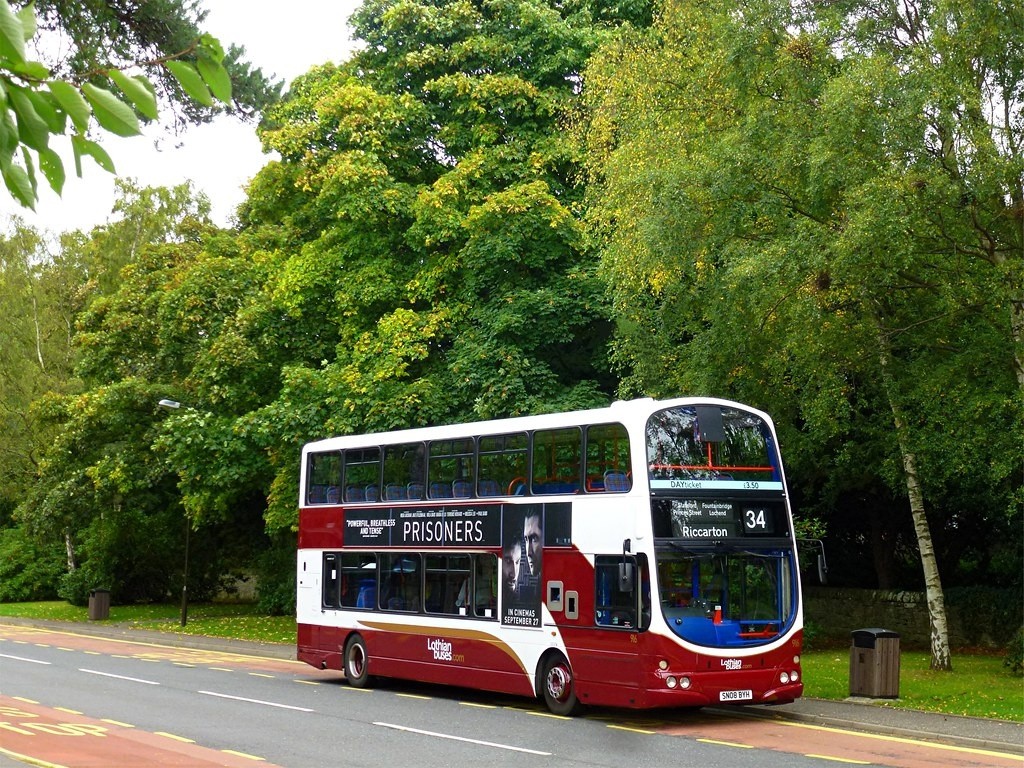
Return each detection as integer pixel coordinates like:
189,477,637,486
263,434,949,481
89,588,111,620
849,627,900,699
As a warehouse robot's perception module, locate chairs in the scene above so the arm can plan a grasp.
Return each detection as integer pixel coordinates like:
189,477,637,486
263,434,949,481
309,469,734,503
344,587,637,627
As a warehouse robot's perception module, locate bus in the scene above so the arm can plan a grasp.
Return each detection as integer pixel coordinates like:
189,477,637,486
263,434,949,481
296,397,830,716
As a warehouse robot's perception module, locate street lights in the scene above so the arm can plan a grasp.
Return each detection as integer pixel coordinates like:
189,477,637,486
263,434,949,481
157,398,205,628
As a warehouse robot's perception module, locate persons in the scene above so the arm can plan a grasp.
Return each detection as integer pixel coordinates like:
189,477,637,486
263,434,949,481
455,562,492,616
524,505,542,576
502,503,523,593
391,555,413,607
674,560,692,581
669,431,714,477
648,564,674,607
343,570,374,606
406,558,441,611
